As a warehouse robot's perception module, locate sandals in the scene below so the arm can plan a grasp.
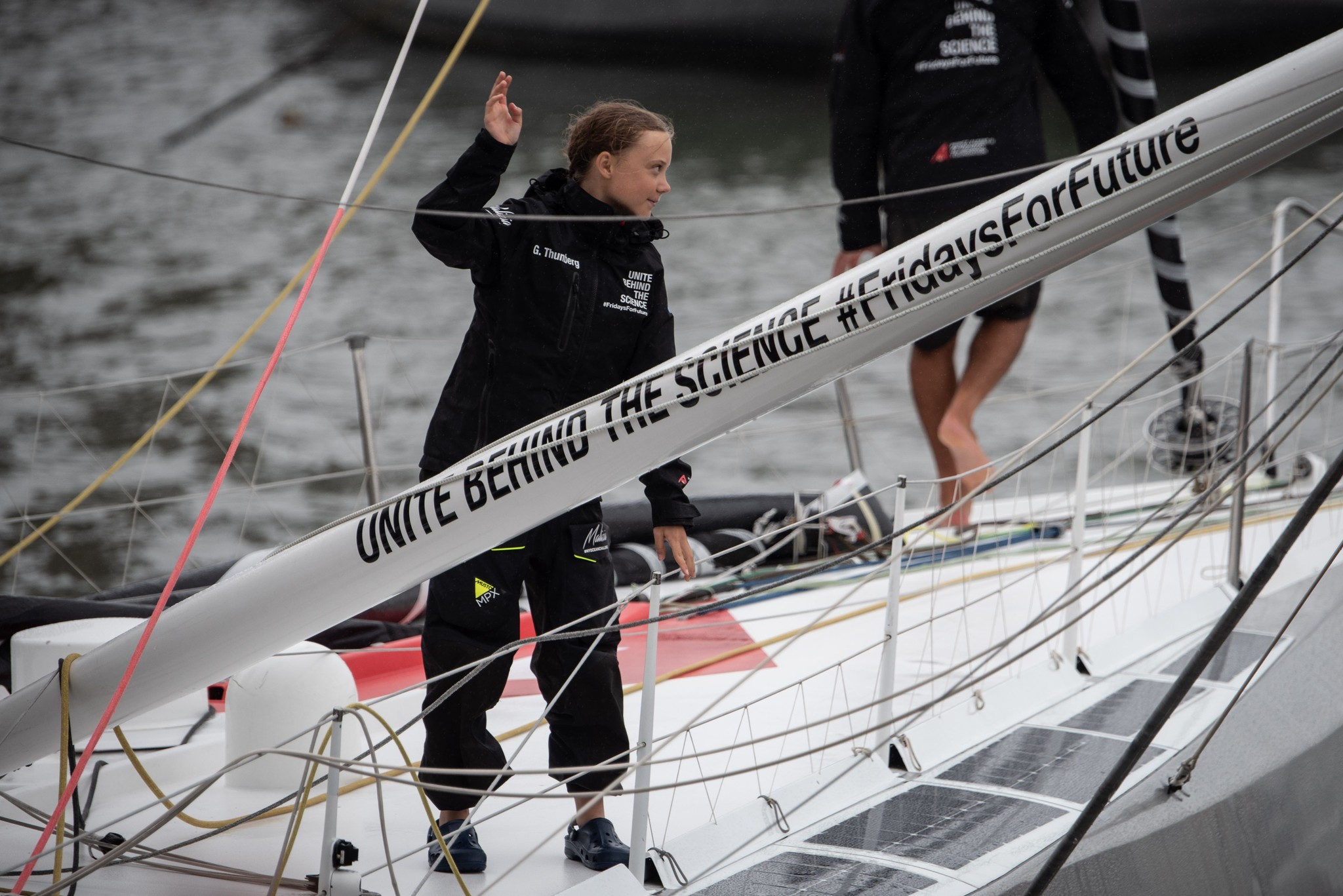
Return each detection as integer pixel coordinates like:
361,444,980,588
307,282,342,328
564,814,631,871
426,818,487,873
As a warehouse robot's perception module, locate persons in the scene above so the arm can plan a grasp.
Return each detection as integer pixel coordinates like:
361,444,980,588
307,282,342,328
413,71,698,877
818,0,1117,527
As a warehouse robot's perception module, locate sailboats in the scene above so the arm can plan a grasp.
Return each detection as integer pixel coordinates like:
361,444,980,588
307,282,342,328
1,27,1343,896
